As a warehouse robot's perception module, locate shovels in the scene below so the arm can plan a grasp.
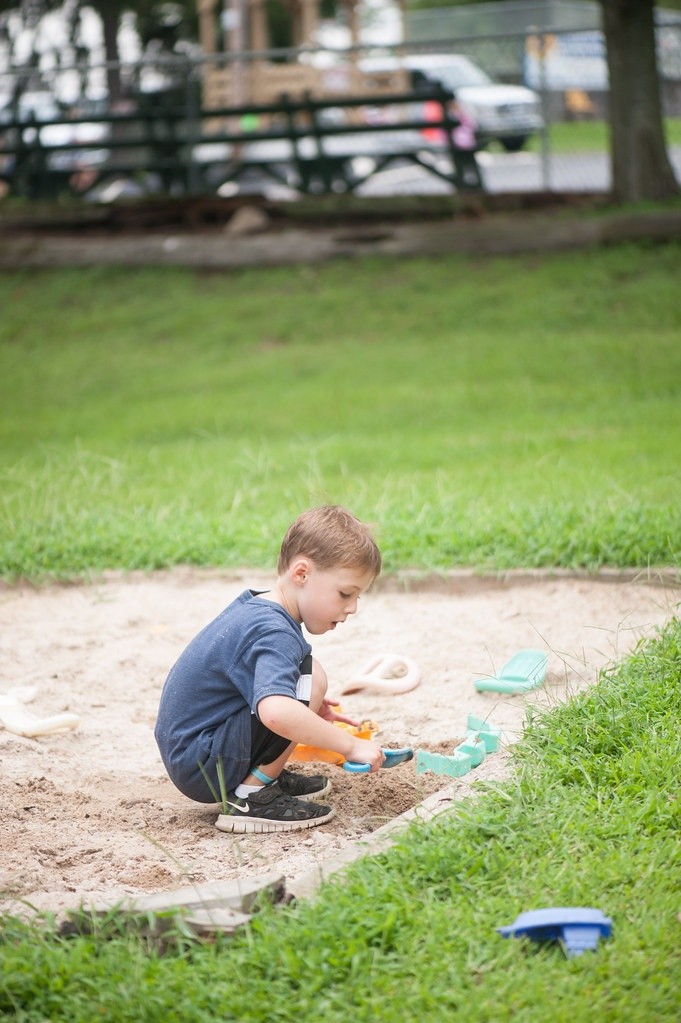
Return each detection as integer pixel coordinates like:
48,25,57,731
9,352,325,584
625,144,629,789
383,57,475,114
342,748,414,773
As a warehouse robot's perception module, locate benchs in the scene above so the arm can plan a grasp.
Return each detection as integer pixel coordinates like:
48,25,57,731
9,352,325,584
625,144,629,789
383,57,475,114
0,71,488,199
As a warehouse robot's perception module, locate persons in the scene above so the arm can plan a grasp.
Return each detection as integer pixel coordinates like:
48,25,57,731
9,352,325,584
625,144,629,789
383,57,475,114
154,504,387,835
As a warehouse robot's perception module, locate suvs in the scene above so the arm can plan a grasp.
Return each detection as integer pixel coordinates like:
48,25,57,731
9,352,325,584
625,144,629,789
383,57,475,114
359,57,548,155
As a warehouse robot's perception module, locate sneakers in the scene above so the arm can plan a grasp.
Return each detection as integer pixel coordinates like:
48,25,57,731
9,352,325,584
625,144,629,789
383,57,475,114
220,769,332,813
215,779,333,833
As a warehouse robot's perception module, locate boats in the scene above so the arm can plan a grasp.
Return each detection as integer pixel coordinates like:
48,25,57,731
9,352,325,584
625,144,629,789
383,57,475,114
495,908,612,962
